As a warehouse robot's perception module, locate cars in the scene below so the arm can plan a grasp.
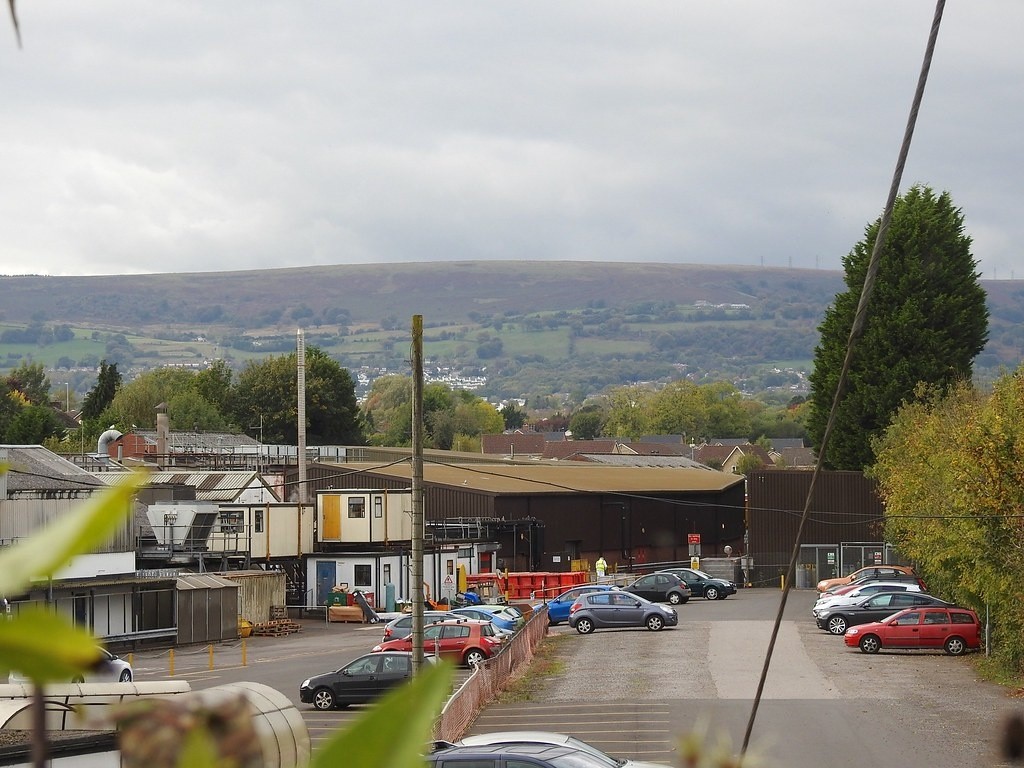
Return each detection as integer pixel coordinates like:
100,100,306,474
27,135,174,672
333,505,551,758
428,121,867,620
371,605,524,672
421,730,675,768
644,567,738,601
531,584,630,627
621,572,691,605
843,606,981,657
568,590,678,634
300,649,445,712
8,645,133,685
817,590,957,636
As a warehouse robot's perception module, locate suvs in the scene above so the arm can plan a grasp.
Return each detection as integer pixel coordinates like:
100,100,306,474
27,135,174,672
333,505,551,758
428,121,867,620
812,562,932,622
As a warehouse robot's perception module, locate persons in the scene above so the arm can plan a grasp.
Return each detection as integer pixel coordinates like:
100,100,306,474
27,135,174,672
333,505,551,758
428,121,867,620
595,556,607,577
